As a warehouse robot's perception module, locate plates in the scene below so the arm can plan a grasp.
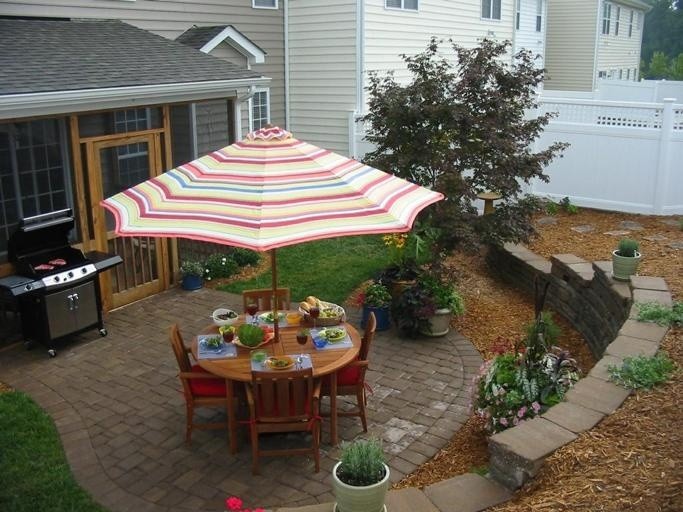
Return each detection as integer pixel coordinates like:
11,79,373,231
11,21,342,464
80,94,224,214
267,354,295,370
199,337,224,354
258,311,284,324
318,329,346,341
218,324,235,335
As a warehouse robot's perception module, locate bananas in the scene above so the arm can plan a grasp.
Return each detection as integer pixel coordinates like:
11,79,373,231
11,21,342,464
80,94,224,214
300,295,319,312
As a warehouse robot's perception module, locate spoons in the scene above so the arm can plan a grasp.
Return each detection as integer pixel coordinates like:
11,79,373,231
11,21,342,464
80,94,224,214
297,355,303,370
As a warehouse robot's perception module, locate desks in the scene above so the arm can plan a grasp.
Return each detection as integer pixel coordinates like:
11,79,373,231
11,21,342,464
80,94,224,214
188,308,365,446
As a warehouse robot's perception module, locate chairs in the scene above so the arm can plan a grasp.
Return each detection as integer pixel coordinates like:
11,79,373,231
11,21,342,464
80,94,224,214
315,310,377,445
236,363,325,474
169,323,245,455
242,288,292,316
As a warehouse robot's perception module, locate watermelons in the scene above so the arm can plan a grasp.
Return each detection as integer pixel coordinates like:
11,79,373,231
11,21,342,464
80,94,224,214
238,324,264,347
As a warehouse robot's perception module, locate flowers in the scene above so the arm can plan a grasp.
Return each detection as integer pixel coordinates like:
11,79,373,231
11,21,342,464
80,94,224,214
460,268,574,448
225,495,264,512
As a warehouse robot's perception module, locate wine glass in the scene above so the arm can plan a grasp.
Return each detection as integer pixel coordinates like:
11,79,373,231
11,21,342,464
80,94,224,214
222,329,234,358
309,306,319,329
296,331,308,357
246,303,257,325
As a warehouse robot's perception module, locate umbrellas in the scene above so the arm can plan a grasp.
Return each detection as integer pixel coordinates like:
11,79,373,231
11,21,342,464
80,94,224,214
98,121,445,344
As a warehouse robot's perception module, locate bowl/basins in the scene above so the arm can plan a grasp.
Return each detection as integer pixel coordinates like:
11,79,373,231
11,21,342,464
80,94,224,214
212,307,240,326
298,301,345,326
312,337,328,348
286,313,300,325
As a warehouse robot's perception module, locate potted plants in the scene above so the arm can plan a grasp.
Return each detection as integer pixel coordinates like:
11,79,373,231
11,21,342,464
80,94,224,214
346,218,468,339
327,427,390,511
611,238,643,280
176,260,211,290
